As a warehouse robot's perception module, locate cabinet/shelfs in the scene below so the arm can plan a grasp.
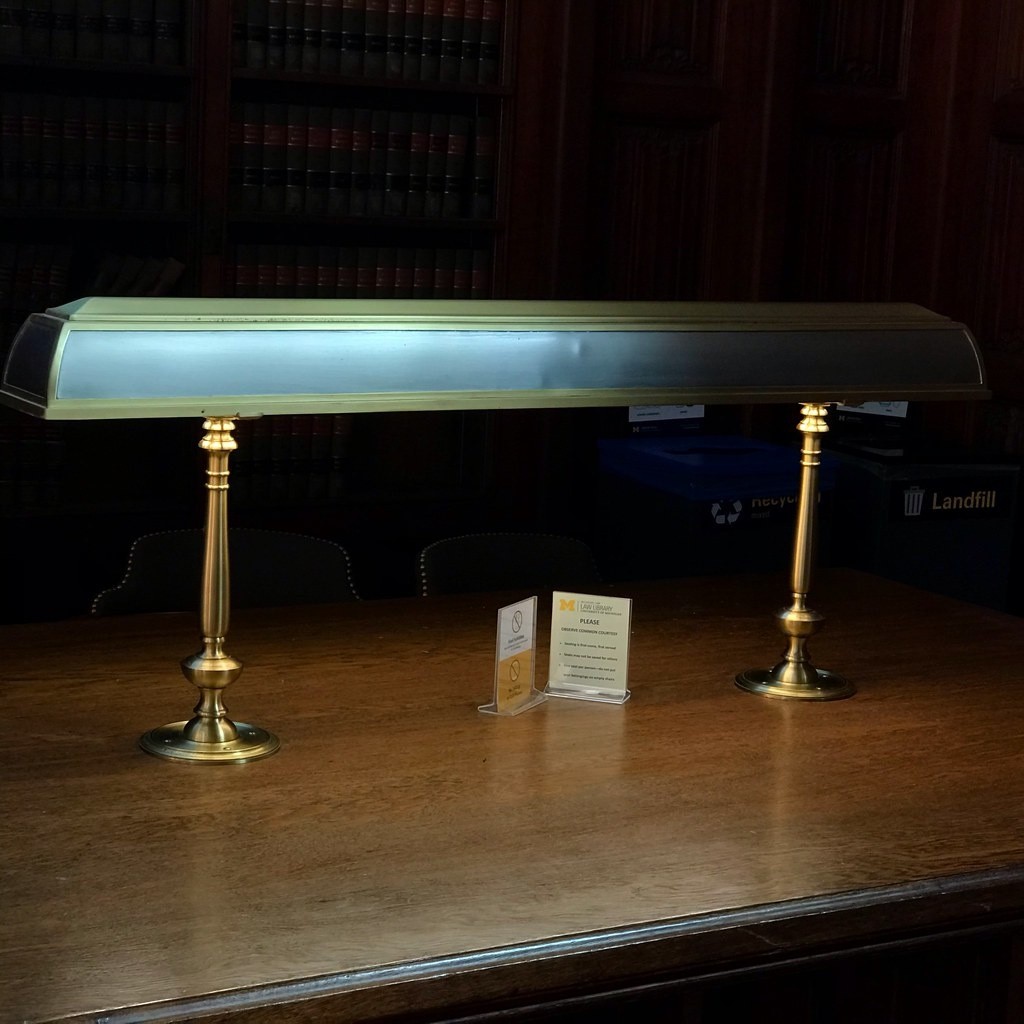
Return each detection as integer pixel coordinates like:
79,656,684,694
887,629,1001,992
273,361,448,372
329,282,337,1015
0,0,551,517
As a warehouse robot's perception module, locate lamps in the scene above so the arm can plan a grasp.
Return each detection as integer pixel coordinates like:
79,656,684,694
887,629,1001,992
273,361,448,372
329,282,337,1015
0,298,985,765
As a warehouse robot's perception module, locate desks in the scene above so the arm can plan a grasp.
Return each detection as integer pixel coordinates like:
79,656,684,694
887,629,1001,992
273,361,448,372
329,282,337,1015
0,564,1024,1024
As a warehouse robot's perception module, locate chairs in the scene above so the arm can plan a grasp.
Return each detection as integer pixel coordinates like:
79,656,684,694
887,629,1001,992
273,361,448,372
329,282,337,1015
91,528,364,619
417,533,606,598
